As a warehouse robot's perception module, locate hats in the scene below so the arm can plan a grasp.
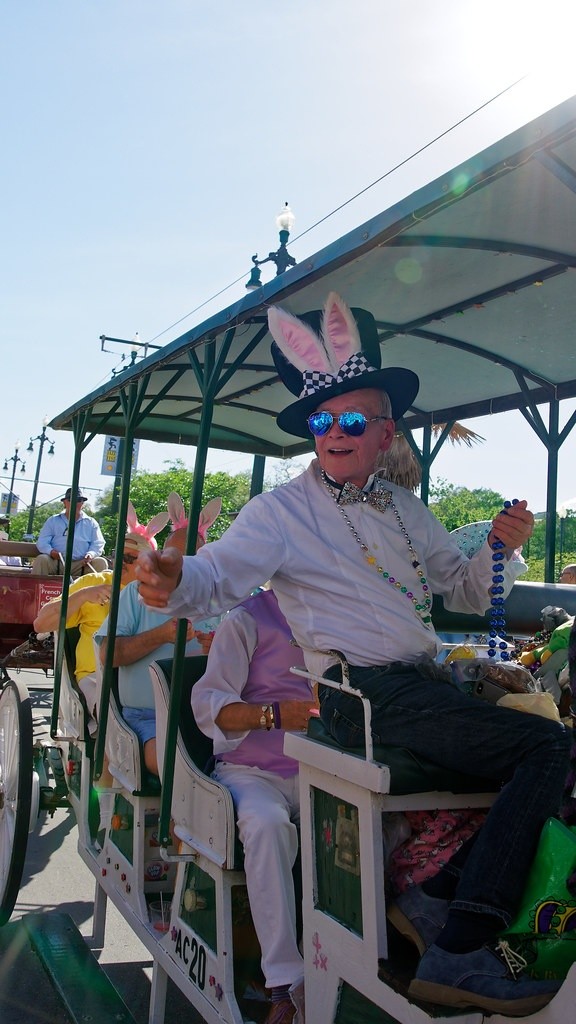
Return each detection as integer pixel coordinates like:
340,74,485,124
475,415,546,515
267,291,420,440
61,488,87,502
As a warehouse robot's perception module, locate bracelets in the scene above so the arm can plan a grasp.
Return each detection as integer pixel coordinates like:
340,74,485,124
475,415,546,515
260,701,281,730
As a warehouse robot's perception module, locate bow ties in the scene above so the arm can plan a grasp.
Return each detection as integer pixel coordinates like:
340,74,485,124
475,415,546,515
337,481,393,514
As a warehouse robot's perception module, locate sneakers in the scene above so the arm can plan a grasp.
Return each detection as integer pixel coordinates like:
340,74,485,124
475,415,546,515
264,998,296,1024
386,885,451,958
408,943,563,1016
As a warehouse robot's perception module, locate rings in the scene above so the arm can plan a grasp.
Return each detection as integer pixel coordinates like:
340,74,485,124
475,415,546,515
104,599,108,602
100,603,104,606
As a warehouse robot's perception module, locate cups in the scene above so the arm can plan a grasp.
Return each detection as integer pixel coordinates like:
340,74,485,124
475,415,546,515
149,902,171,933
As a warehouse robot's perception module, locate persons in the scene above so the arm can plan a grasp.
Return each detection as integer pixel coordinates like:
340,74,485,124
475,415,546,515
560,564,576,584
33,501,170,854
94,491,221,848
31,488,108,576
191,581,320,1024
137,308,570,1014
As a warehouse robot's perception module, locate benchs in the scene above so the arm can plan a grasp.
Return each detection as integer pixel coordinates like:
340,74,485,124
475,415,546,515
0,541,43,576
53,619,576,1024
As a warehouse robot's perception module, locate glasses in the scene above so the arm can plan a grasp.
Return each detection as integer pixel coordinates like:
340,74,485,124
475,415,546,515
306,411,387,437
123,553,138,564
68,498,82,503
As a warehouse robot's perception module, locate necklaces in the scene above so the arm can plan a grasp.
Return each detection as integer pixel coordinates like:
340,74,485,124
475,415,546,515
489,499,519,659
321,468,433,629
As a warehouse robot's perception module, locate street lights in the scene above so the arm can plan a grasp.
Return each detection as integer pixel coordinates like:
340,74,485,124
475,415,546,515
27,415,56,542
2,442,27,536
558,506,567,574
